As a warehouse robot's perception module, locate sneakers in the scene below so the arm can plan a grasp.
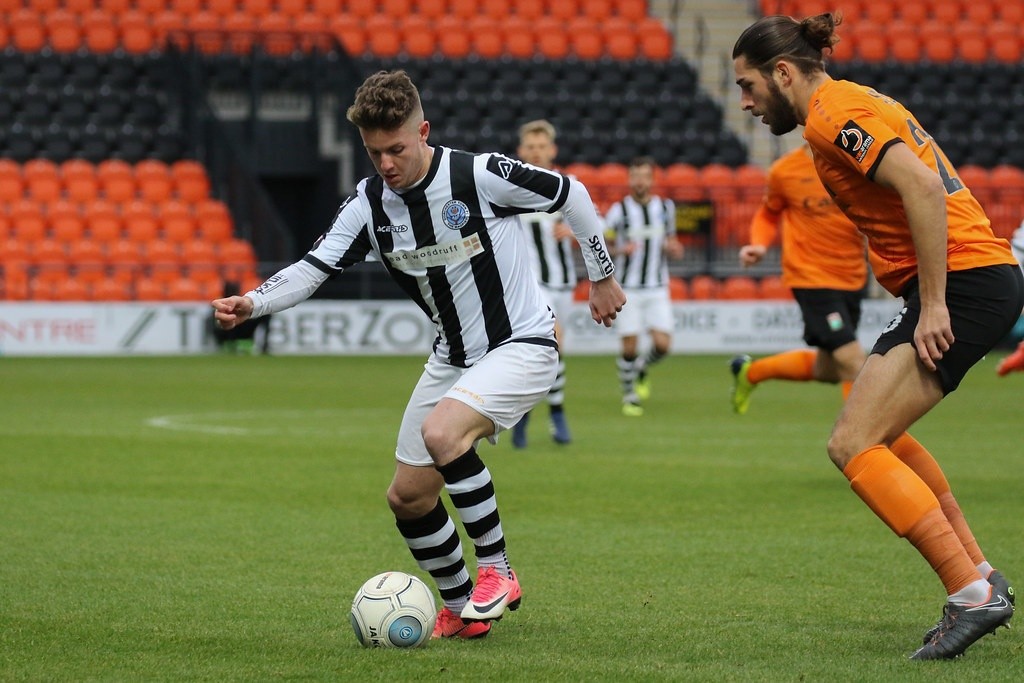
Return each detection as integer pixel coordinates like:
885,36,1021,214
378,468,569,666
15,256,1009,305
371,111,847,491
549,414,570,444
623,401,644,416
924,569,1015,646
911,584,1015,659
429,608,490,640
730,354,755,415
637,374,649,400
513,423,528,450
996,341,1024,377
461,566,522,624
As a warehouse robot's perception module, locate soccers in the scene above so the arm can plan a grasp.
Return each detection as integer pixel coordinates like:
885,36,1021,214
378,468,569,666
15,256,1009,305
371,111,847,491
351,571,437,650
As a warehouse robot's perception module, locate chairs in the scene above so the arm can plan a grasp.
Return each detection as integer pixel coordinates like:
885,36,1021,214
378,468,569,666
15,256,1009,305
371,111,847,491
0,0,1024,300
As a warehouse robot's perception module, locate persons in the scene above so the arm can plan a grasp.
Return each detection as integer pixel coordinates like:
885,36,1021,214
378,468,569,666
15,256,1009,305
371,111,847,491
513,120,609,449
730,141,869,415
605,155,685,416
732,13,1024,661
998,214,1024,377
210,70,627,635
